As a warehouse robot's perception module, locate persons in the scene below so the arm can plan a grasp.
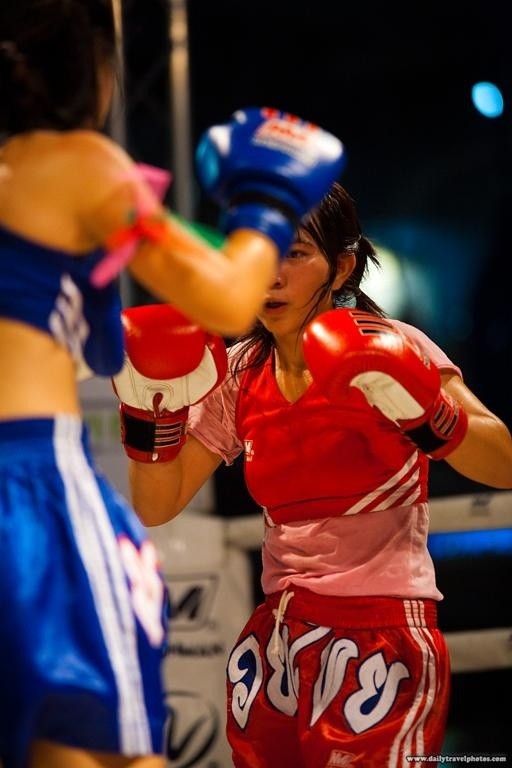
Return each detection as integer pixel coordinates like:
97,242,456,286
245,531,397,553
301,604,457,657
112,182,512,768
0,0,349,768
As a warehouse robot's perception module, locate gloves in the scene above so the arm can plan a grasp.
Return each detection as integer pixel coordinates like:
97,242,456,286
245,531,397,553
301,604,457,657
98,300,236,465
194,103,349,261
299,305,470,464
